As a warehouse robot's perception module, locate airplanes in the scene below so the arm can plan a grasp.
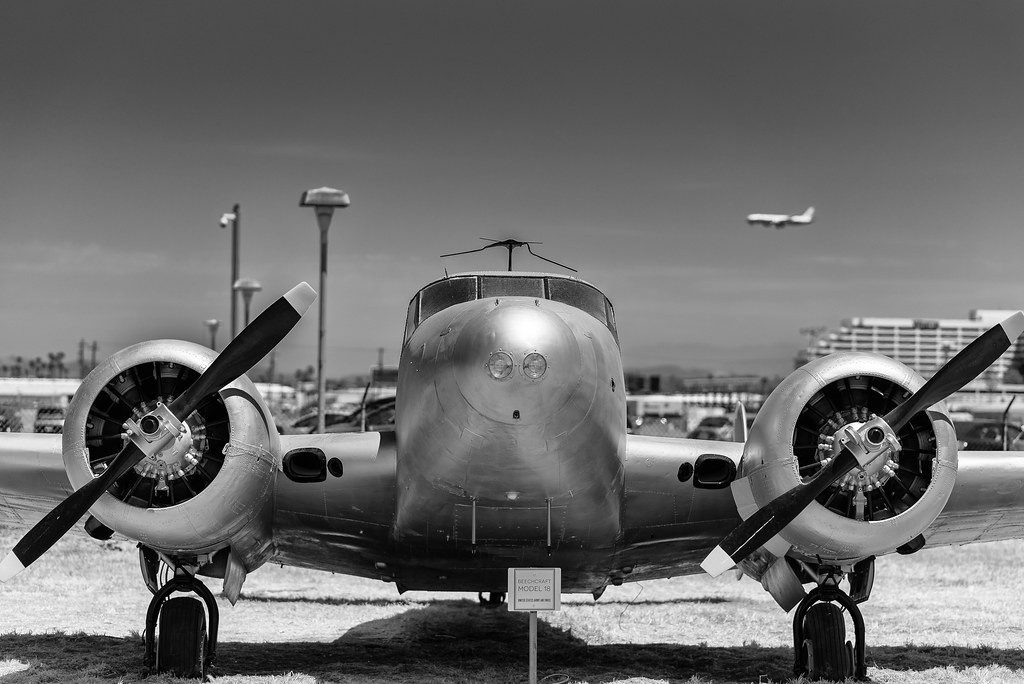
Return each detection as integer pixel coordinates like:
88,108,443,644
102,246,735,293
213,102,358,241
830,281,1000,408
1,236,1024,678
744,207,816,231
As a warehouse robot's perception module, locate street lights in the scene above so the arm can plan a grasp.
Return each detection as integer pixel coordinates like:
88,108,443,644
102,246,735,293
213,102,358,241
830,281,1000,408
300,186,351,434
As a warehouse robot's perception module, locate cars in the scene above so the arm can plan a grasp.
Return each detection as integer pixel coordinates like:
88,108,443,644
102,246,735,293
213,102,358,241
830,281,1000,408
33,407,65,432
687,417,734,440
